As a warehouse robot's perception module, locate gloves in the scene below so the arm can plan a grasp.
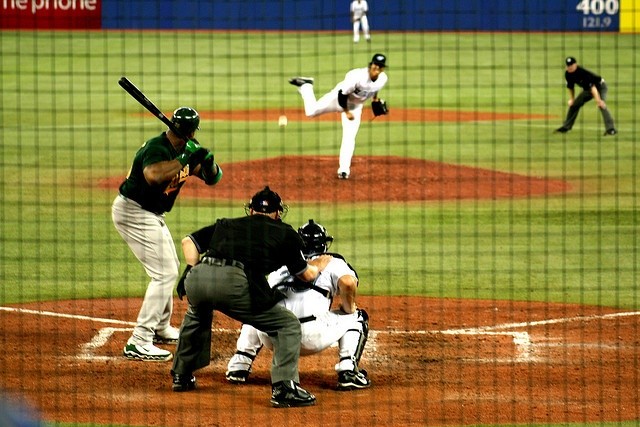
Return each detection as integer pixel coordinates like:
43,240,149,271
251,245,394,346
189,147,215,175
176,138,203,167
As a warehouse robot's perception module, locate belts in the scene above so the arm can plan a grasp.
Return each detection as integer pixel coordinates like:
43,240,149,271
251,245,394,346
298,314,317,323
201,256,245,270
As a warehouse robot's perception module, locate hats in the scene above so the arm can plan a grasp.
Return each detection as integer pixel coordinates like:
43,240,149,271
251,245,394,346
566,57,576,65
372,54,388,67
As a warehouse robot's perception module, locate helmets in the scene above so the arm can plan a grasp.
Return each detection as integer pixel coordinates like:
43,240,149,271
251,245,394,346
242,186,290,221
297,219,334,255
171,107,200,131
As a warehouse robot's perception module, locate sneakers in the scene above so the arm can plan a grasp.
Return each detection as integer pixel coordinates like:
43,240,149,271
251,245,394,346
288,77,314,86
269,380,316,407
338,172,349,179
604,129,616,136
557,128,567,132
336,370,372,390
122,335,173,361
172,375,196,392
225,370,246,383
153,322,180,344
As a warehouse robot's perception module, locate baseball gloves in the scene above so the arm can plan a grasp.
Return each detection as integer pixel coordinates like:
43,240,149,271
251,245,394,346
372,101,387,116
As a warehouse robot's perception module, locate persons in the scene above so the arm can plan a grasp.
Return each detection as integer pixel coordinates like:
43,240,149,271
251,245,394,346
552,57,617,136
288,52,389,180
226,218,372,389
171,186,333,408
110,107,222,361
348,0,372,43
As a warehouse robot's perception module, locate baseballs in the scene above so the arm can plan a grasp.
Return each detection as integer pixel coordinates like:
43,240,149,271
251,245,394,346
279,116,287,127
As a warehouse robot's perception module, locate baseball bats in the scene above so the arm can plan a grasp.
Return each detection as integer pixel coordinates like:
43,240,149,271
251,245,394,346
118,77,189,143
